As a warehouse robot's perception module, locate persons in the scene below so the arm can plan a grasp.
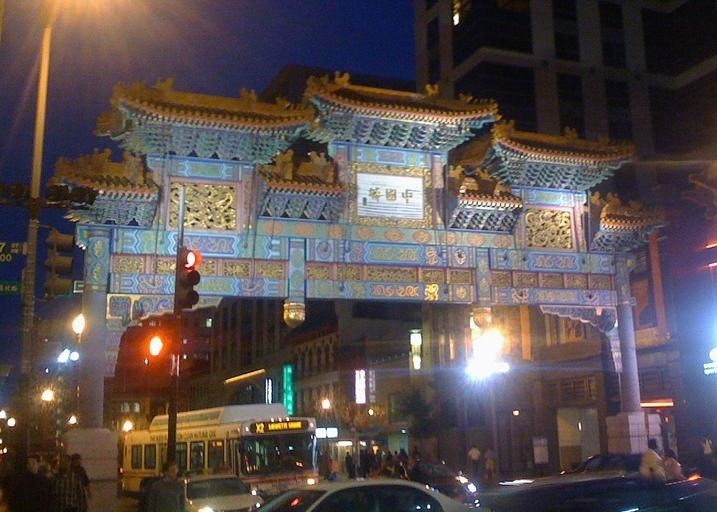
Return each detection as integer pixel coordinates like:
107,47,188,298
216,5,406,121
662,450,685,480
144,459,189,511
345,446,421,479
640,436,665,481
0,451,92,512
699,432,713,474
467,444,481,480
483,444,497,481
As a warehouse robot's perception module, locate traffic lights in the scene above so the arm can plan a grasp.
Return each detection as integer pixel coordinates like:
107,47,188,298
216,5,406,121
44,229,76,299
147,245,201,361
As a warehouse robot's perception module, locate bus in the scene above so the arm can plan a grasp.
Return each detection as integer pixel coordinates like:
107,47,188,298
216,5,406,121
124,403,318,497
